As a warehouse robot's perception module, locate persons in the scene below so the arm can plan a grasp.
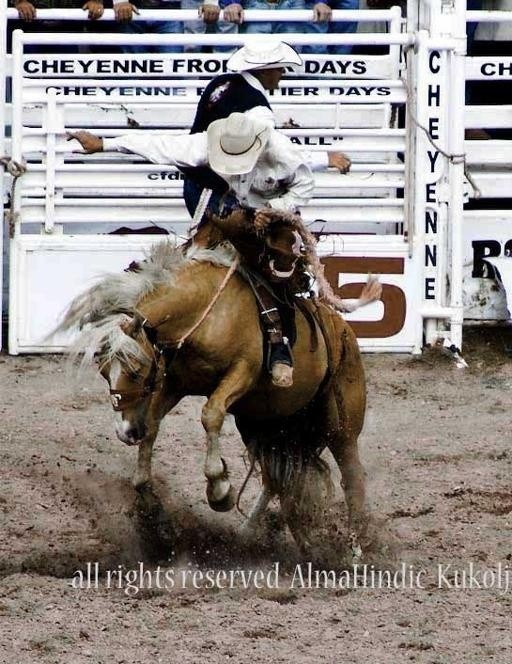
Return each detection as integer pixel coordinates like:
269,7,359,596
12,0,407,81
182,37,353,228
62,106,315,389
467,0,512,139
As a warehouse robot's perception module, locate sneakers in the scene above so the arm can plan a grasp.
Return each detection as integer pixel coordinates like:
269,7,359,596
271,361,293,387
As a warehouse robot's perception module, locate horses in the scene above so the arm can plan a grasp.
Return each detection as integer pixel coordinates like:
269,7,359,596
44,229,382,579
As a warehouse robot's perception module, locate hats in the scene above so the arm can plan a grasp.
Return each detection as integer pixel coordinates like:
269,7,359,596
226,38,302,72
207,105,275,175
